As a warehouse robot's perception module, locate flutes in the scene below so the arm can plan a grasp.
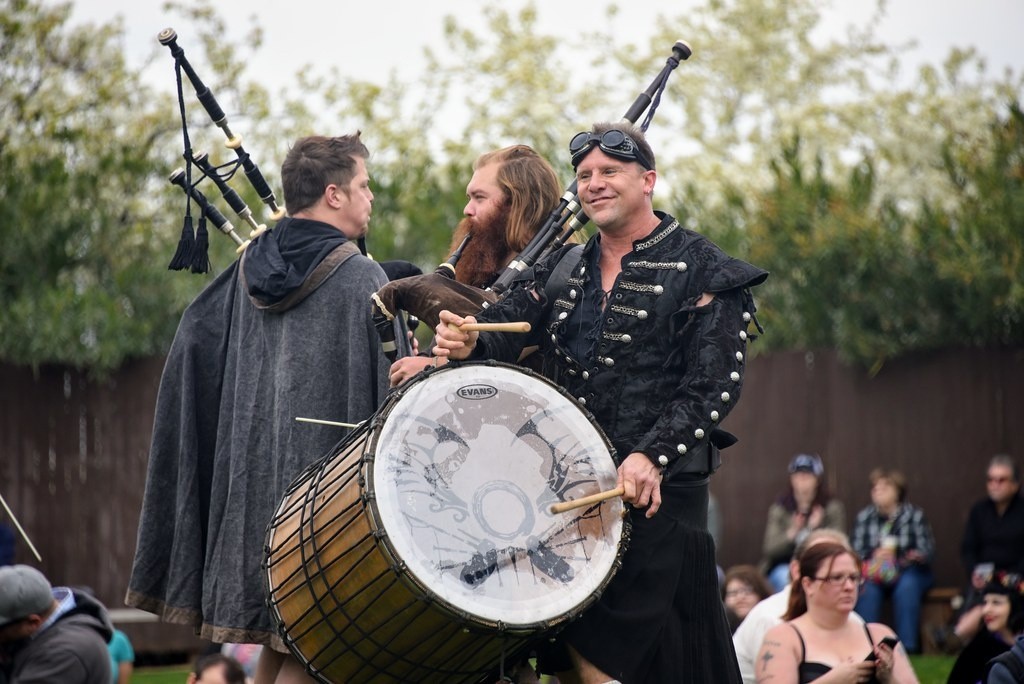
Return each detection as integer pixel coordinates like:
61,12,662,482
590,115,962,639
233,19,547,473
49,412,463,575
405,39,694,356
154,26,374,264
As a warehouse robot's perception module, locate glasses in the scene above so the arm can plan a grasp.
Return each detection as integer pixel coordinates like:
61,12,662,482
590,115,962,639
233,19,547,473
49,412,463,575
986,477,1009,485
569,131,652,173
815,574,865,586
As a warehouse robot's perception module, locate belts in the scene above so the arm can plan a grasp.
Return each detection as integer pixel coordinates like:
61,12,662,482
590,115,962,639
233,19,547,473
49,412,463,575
679,446,722,474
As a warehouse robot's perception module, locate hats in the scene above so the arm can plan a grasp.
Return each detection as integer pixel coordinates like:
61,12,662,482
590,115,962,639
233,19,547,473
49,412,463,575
0,565,54,624
788,453,822,476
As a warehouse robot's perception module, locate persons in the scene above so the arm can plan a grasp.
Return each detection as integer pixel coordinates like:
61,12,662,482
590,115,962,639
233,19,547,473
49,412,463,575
755,542,922,684
124,132,420,684
720,565,777,634
70,583,135,684
731,527,852,684
0,564,115,684
980,572,1024,684
442,122,770,684
849,465,941,655
954,452,1024,643
370,145,597,684
186,653,246,684
760,452,851,596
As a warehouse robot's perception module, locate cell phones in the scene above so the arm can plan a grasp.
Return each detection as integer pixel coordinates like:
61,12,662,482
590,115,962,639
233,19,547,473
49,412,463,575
865,637,898,661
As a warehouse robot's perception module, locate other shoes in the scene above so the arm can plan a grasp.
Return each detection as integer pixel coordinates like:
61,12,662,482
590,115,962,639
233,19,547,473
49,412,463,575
926,626,962,653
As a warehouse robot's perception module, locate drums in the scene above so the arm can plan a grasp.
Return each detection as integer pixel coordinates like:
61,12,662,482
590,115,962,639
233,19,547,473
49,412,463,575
260,358,636,684
